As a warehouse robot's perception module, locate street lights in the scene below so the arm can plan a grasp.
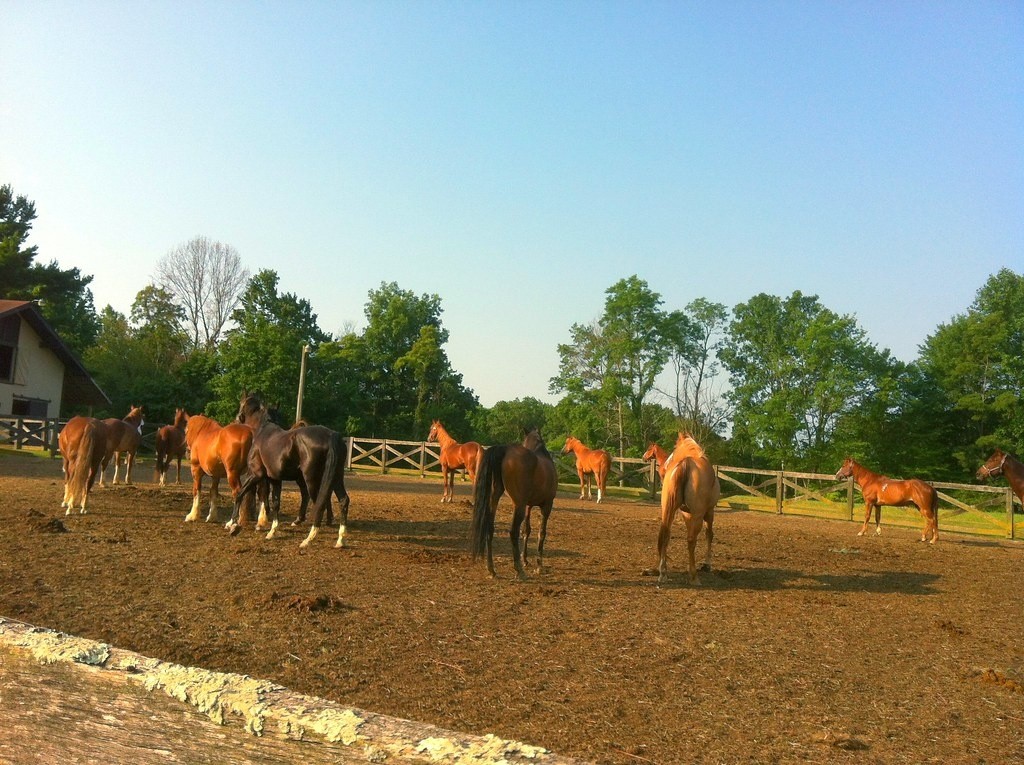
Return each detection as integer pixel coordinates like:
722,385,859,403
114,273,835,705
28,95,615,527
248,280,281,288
296,344,311,421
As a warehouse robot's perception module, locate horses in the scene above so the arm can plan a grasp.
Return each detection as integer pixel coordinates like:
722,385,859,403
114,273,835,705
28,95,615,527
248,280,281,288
836,458,940,545
183,412,253,531
229,386,350,549
642,443,669,481
561,436,611,504
99,405,145,488
59,416,107,516
658,431,720,587
427,418,485,503
472,426,559,580
154,407,187,488
975,448,1024,510
